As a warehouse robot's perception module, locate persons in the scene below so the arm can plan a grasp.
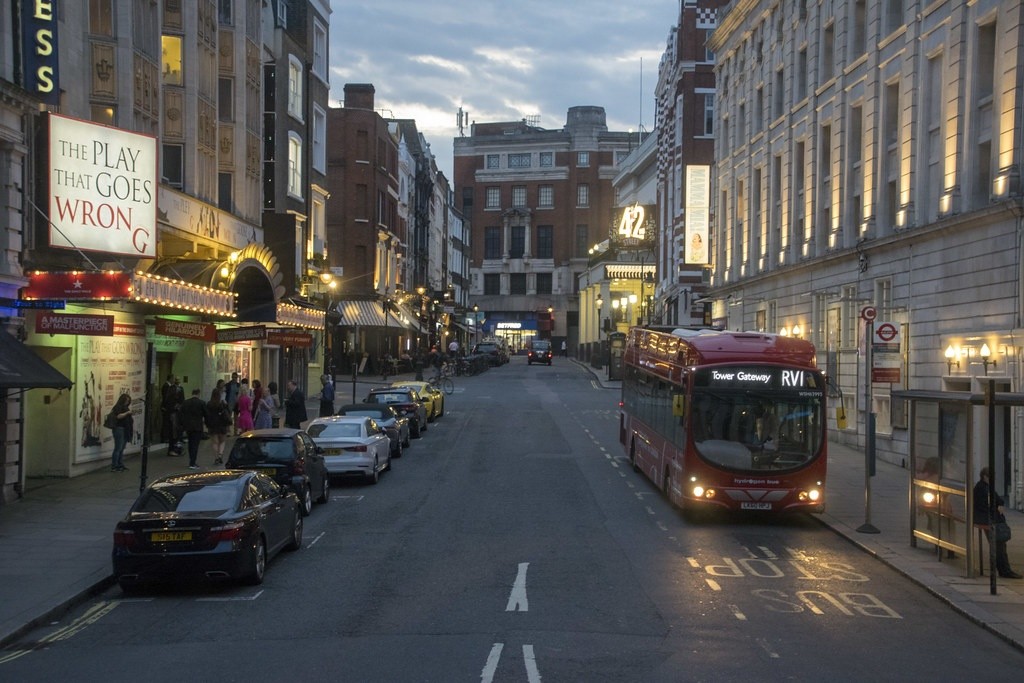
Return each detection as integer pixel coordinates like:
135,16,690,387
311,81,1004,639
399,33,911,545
160,374,280,469
449,338,459,356
558,339,568,358
283,380,308,429
916,456,958,559
110,394,134,473
320,375,335,417
973,467,1024,579
431,345,444,384
401,349,412,360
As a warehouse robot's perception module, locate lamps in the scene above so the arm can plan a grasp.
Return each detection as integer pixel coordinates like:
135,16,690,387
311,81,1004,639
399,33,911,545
979,343,997,376
944,345,959,376
780,325,803,339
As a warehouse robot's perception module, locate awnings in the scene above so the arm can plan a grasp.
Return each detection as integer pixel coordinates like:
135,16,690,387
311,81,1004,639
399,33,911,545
454,322,475,333
329,301,408,329
0,325,73,389
394,302,432,334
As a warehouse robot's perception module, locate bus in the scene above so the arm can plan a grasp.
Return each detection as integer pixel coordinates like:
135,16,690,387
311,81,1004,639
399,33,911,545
618,324,848,513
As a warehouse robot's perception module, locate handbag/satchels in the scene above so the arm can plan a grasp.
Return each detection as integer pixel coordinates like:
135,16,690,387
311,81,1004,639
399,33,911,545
992,522,1011,544
104,413,114,429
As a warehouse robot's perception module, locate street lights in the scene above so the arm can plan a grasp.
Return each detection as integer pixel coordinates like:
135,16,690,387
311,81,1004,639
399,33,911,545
414,284,428,381
317,259,335,374
473,301,479,343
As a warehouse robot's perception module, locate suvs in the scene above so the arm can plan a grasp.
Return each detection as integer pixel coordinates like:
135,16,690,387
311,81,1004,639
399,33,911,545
472,341,503,367
528,340,553,366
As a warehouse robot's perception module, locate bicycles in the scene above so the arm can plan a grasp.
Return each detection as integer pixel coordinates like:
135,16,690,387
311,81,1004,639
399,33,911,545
439,353,475,378
427,369,455,395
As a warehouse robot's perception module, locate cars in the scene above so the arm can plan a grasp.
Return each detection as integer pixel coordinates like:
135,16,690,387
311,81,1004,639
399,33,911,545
303,379,445,486
112,470,304,594
224,428,331,516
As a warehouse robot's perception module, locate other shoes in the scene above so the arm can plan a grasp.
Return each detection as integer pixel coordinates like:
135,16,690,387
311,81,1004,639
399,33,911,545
1001,571,1023,579
119,466,129,471
188,464,200,470
110,468,116,472
214,457,223,465
167,450,178,456
